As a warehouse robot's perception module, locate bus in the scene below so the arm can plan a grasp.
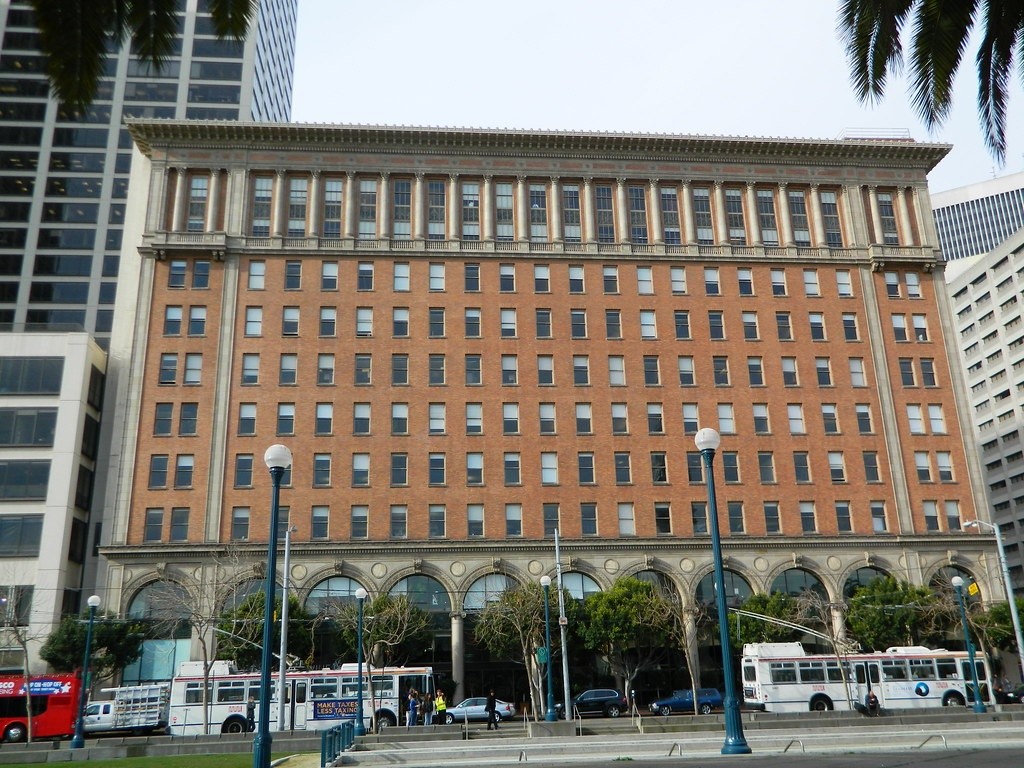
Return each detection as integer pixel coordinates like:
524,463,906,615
0,669,83,743
165,618,446,735
727,605,998,713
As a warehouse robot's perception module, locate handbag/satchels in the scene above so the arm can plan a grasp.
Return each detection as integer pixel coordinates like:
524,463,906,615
485,706,489,711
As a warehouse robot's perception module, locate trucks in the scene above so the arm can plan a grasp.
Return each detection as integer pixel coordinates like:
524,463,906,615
82,680,172,737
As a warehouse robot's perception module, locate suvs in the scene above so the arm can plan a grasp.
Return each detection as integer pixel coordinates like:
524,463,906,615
553,688,628,720
648,688,723,717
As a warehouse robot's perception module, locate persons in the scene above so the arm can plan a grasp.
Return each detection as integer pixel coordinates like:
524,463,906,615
865,690,880,717
405,687,422,726
484,690,502,730
1001,672,1012,691
434,689,447,725
246,695,256,732
422,693,433,725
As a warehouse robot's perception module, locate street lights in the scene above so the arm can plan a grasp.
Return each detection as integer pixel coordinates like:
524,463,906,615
950,574,986,714
693,427,755,755
540,574,559,722
69,593,102,748
354,587,368,736
250,443,293,768
962,519,1024,673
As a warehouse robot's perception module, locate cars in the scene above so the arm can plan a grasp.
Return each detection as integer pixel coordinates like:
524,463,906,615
446,695,518,725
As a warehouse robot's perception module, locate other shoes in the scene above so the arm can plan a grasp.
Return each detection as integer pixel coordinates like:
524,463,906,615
487,728,494,730
495,726,502,730
